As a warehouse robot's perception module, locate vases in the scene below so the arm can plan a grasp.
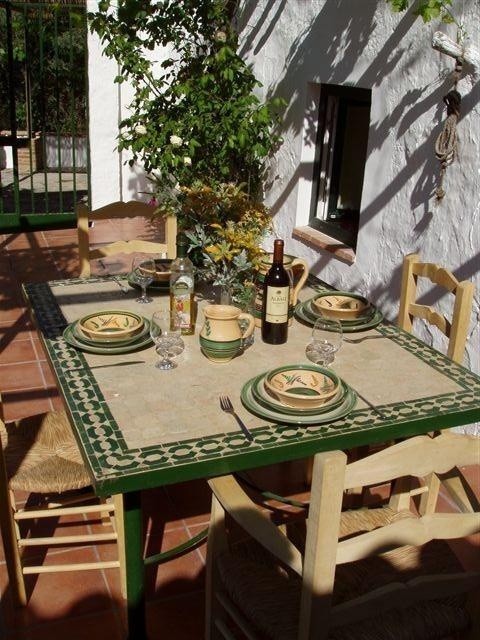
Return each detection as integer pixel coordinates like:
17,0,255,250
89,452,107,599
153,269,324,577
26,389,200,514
214,284,232,305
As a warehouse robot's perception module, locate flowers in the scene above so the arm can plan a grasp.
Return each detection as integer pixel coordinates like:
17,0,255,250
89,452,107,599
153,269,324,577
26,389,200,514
185,220,270,294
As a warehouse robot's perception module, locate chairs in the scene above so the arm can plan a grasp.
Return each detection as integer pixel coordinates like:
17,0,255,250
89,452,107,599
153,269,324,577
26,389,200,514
203,432,480,640
388,256,480,514
76,199,177,278
0,395,128,601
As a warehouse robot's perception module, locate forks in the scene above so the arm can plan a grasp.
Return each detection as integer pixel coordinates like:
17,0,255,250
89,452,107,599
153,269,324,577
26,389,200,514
341,334,399,345
219,396,253,443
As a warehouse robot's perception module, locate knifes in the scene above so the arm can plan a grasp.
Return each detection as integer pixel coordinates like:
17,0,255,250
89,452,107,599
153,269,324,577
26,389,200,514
111,275,129,295
66,360,145,371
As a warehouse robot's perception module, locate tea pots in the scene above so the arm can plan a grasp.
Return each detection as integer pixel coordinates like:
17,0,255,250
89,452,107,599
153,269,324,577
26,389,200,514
199,304,255,362
255,255,309,329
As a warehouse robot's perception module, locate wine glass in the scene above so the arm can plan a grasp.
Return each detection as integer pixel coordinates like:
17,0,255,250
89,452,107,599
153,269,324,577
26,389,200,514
150,311,181,370
312,318,343,368
131,256,156,304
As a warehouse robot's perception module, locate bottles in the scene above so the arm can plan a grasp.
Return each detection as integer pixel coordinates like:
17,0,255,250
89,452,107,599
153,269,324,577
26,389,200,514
170,235,195,335
263,238,290,344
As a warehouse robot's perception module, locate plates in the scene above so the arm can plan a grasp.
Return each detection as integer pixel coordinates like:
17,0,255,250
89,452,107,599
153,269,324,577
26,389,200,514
241,363,357,424
64,311,160,353
295,290,384,332
128,260,174,290
